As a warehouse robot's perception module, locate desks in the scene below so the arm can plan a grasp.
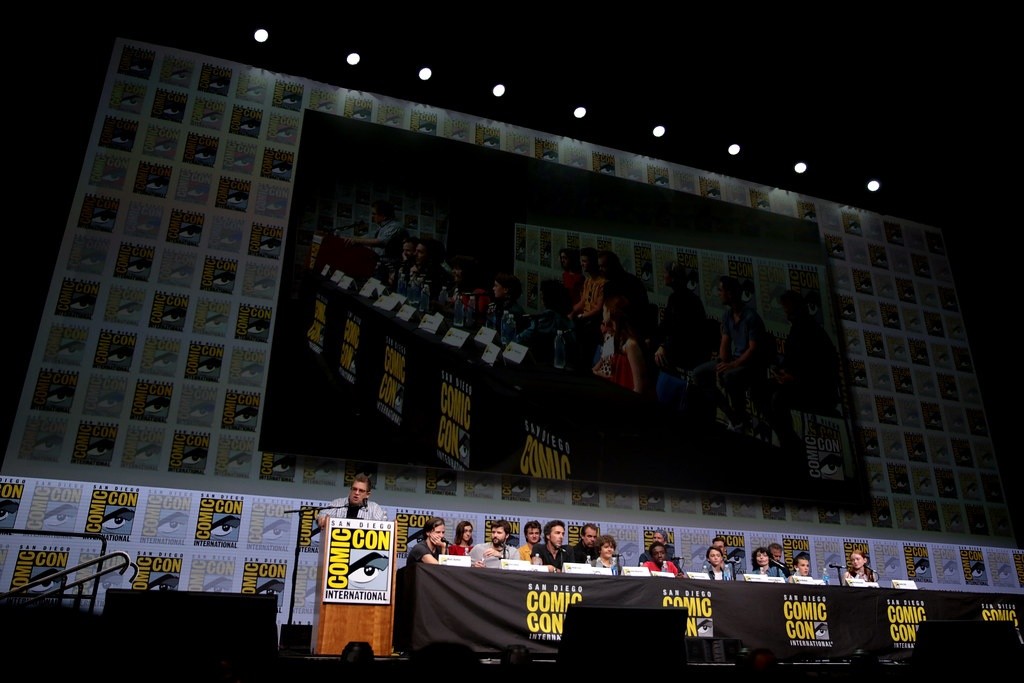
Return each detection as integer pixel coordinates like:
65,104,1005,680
270,261,844,501
392,563,1024,664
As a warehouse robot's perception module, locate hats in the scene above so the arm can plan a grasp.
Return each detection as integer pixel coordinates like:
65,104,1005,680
664,261,687,280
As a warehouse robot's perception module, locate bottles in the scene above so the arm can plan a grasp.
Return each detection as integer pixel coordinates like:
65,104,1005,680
701,565,709,573
822,568,831,585
662,561,668,572
463,548,470,556
794,568,801,576
554,330,566,369
479,554,486,567
723,565,731,581
610,557,619,576
532,553,543,565
585,555,592,565
595,557,603,567
640,562,644,567
397,274,516,345
758,567,765,574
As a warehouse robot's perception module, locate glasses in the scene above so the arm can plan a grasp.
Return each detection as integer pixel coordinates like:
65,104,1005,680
371,211,385,216
350,487,370,493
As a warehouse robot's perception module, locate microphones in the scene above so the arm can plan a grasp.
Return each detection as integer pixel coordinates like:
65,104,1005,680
344,219,364,230
501,540,505,546
463,292,489,296
864,564,876,573
362,497,368,508
829,563,844,569
770,558,784,569
612,553,623,557
555,546,567,553
724,560,737,564
441,538,453,546
671,556,684,560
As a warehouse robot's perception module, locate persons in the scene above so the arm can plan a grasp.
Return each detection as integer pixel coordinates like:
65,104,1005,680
469,520,520,568
517,520,543,561
317,473,389,527
344,202,841,470
638,530,673,568
406,517,449,567
638,542,686,578
572,523,600,563
449,521,475,556
841,550,876,586
591,535,617,568
530,520,576,573
751,543,791,583
700,537,726,573
706,547,734,581
788,555,810,584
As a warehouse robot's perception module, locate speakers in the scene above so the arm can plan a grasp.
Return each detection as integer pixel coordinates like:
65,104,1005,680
554,603,689,668
100,588,279,663
